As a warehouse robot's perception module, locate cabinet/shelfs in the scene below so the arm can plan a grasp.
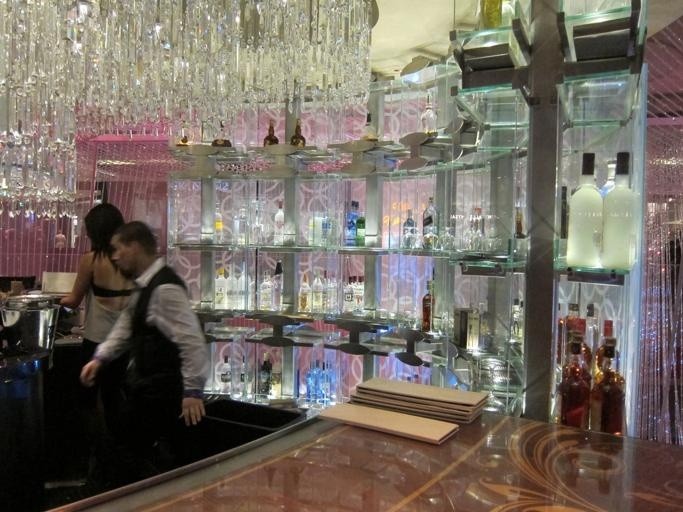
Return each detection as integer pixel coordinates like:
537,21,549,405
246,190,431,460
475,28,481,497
164,66,649,436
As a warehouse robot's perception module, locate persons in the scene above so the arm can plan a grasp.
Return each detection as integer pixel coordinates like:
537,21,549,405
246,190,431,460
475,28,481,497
79,221,209,499
59,204,137,490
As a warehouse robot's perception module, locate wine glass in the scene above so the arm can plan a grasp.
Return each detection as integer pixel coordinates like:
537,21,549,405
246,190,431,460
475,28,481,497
422,225,439,249
438,226,455,250
402,227,417,248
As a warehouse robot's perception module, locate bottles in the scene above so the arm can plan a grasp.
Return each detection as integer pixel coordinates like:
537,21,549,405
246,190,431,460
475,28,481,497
308,211,322,246
313,268,323,309
210,120,232,146
345,201,359,246
512,213,527,239
360,112,377,139
297,273,312,313
422,196,439,249
564,303,586,341
471,207,486,251
421,280,434,332
556,303,563,364
583,303,600,350
238,260,252,311
509,298,524,340
305,358,337,401
601,151,642,271
257,352,271,395
216,268,227,309
402,208,417,248
239,356,253,392
221,355,232,391
227,261,238,311
342,275,365,313
289,118,306,147
262,118,279,147
419,88,436,134
215,203,222,243
556,342,590,432
566,151,603,268
273,201,285,246
324,271,338,309
355,212,367,246
591,344,627,440
596,319,622,376
601,158,618,192
259,271,274,309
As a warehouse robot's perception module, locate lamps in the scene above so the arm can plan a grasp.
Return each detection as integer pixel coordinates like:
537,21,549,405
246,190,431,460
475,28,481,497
0,72,79,223
0,2,374,127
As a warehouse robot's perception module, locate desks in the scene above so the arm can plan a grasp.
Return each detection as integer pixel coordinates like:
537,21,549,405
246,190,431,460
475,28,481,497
37,400,683,510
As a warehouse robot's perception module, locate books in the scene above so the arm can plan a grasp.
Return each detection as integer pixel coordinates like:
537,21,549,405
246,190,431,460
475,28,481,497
316,375,489,443
